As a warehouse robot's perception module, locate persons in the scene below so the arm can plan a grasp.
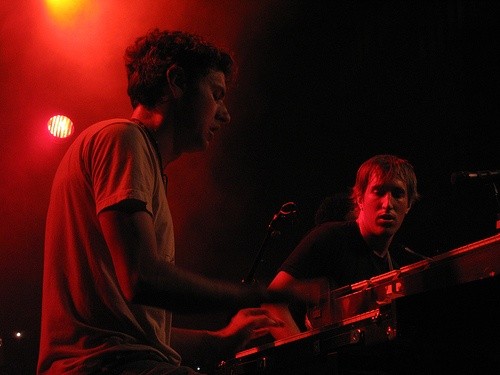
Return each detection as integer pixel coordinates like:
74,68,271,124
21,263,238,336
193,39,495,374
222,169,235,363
33,27,335,375
266,150,441,372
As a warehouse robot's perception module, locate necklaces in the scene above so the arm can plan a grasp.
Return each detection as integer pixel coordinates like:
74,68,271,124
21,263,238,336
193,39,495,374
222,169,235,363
130,117,170,197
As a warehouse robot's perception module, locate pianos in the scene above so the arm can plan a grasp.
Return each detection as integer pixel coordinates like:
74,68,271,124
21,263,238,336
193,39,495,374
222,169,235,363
210,233,500,375
